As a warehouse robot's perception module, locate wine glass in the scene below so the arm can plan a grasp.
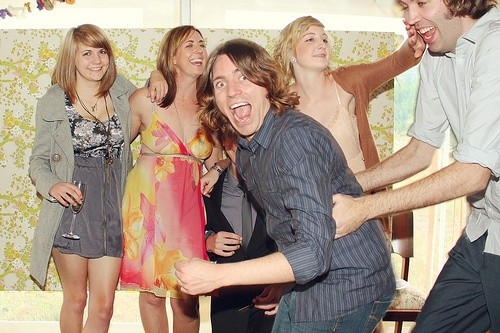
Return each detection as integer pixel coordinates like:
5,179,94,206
62,180,87,239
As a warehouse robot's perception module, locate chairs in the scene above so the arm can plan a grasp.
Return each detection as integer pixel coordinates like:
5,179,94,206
380,211,426,333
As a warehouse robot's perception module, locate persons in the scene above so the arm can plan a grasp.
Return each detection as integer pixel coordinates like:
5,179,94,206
200,15,426,243
28,23,168,332
173,37,397,332
128,25,224,333
202,119,296,333
332,0,500,332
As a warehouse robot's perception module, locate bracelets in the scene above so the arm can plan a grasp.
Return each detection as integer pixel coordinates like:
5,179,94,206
212,163,223,173
